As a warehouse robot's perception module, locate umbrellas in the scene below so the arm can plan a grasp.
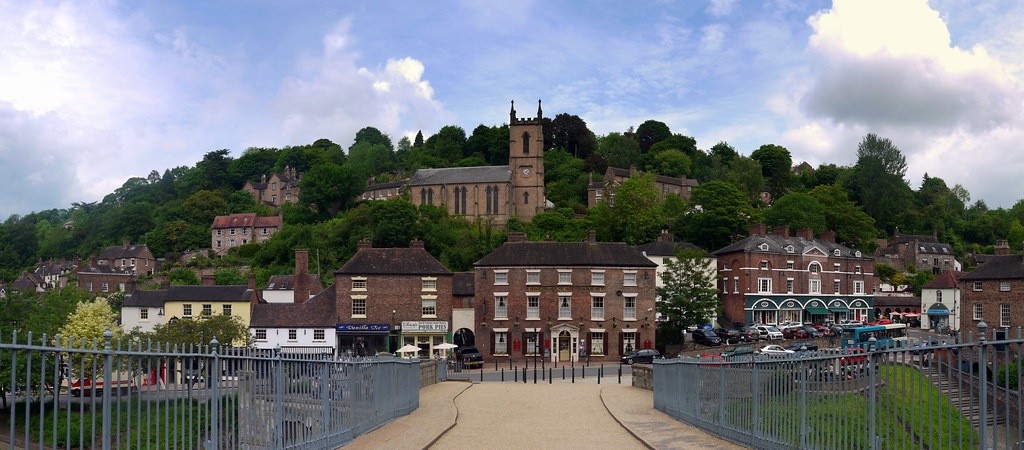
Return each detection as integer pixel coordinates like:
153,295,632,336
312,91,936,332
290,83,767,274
434,342,458,357
395,343,422,352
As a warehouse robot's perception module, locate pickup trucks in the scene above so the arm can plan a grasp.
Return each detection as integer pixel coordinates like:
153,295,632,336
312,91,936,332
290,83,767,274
459,346,484,368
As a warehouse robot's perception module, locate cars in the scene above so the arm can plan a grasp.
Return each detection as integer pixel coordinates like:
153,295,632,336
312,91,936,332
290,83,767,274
760,345,794,358
870,318,892,326
787,341,818,353
715,328,736,338
620,348,661,365
184,370,200,383
692,328,721,345
835,348,871,364
699,323,715,331
768,318,865,342
911,339,940,353
720,346,755,358
688,325,697,331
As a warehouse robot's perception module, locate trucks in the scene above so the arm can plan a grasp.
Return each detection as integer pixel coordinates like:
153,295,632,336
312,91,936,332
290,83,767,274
67,368,136,398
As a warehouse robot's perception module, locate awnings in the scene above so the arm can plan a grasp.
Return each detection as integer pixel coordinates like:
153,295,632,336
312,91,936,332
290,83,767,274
280,345,333,354
807,308,832,315
830,307,851,312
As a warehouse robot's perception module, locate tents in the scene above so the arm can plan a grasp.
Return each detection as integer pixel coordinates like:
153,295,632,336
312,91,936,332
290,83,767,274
889,310,921,322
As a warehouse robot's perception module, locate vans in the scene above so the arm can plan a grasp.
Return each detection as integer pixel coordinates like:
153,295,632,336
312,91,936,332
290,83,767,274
758,326,784,341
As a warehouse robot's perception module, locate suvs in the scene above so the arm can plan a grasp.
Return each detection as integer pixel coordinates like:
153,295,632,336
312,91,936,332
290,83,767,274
737,327,760,340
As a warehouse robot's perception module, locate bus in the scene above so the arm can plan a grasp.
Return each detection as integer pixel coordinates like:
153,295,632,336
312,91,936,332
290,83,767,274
840,324,909,350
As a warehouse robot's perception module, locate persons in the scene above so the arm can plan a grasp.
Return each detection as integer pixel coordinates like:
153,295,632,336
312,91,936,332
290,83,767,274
696,352,700,359
308,355,371,400
677,354,681,357
661,355,665,360
